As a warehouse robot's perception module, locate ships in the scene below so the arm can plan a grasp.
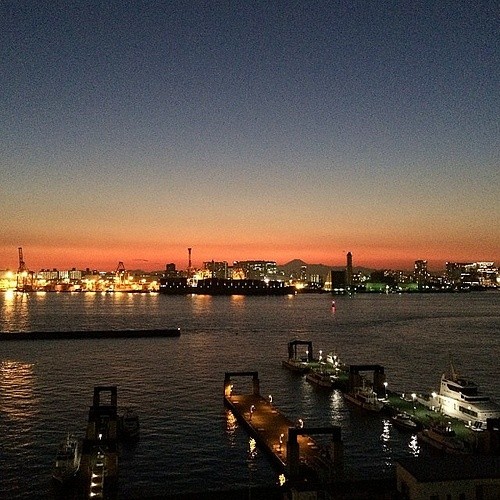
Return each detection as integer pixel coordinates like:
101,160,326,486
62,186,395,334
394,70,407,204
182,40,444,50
156,277,298,295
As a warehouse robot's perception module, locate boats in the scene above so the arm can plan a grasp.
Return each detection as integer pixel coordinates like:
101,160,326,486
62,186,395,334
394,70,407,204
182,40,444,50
416,423,470,456
306,368,334,388
282,357,309,372
411,359,500,431
391,412,426,433
51,430,81,483
343,378,385,411
